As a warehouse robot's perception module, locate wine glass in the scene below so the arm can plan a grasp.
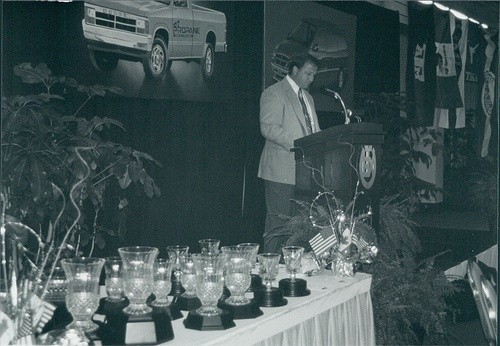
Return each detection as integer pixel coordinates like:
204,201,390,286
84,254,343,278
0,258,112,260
282,245,305,283
257,252,282,292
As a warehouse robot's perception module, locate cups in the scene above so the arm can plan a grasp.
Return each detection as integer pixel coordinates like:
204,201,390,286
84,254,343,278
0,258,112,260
102,255,126,303
117,244,159,315
58,256,106,333
191,252,226,317
236,242,261,271
166,236,224,299
149,258,173,308
220,246,255,306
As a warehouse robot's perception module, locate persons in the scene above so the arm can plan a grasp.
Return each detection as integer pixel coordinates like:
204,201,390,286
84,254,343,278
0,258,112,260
257,53,322,253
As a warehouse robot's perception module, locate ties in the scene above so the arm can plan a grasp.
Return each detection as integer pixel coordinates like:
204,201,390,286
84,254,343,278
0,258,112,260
299,89,312,136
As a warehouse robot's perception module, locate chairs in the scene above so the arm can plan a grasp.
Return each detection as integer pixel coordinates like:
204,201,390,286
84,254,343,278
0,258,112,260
467,257,498,346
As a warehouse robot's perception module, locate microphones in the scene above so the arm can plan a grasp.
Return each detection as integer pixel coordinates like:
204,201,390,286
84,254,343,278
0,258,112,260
325,88,350,124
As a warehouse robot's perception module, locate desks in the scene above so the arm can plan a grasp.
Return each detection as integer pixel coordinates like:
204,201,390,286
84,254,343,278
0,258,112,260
92,263,373,346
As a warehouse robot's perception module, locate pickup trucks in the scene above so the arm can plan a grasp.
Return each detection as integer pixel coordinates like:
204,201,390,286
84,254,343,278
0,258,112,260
82,1,228,82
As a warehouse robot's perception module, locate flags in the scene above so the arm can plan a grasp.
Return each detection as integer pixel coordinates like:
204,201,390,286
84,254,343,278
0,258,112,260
406,0,498,158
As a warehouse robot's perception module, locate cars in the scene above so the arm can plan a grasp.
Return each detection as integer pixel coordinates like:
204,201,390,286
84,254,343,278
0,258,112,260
271,18,350,93
